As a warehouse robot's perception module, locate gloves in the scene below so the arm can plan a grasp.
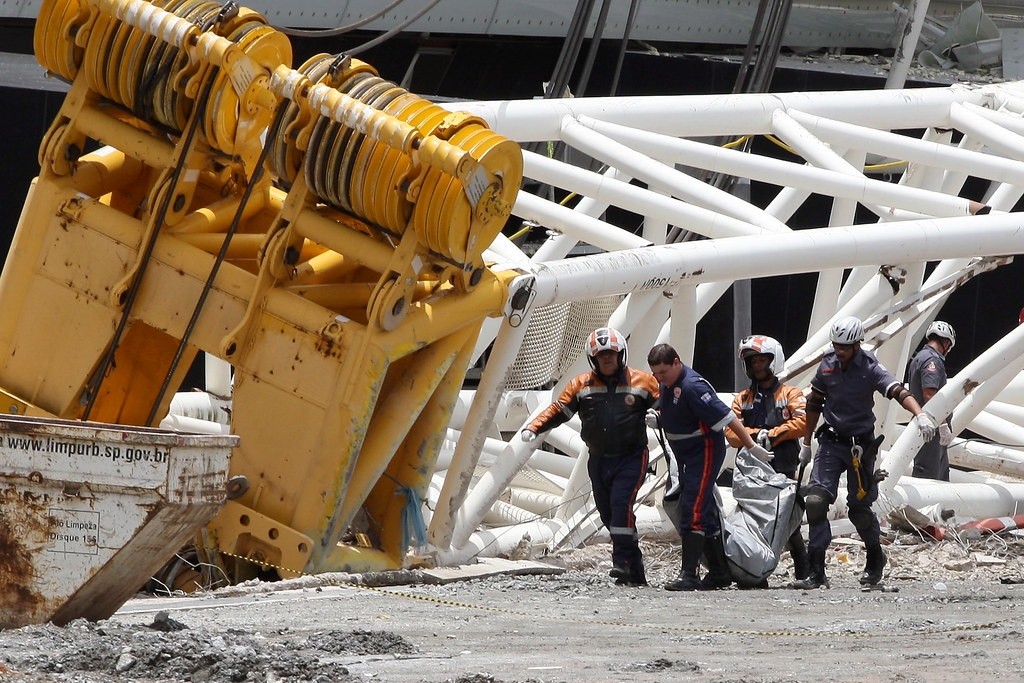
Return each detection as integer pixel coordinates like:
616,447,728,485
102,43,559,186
749,443,775,463
798,443,812,467
645,408,662,428
938,423,952,446
916,413,936,443
521,429,537,442
756,429,772,451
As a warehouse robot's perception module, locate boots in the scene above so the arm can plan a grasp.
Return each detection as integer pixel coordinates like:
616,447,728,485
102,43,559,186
700,533,732,589
665,529,705,590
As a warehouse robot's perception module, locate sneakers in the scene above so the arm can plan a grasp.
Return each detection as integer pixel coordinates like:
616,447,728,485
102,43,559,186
788,572,831,588
860,553,887,583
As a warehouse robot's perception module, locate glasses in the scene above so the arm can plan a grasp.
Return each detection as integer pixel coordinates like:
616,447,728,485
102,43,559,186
596,350,616,358
750,355,772,364
832,343,852,351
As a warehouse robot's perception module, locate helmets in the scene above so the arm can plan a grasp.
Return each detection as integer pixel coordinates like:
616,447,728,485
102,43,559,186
585,328,628,374
829,317,864,344
926,321,956,352
737,335,785,379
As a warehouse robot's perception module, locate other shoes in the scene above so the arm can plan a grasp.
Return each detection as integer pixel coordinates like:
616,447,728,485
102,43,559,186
609,560,648,586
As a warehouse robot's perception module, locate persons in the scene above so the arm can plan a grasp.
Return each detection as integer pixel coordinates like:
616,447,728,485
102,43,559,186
722,336,808,589
909,321,956,480
520,326,662,587
790,316,935,587
648,340,774,591
